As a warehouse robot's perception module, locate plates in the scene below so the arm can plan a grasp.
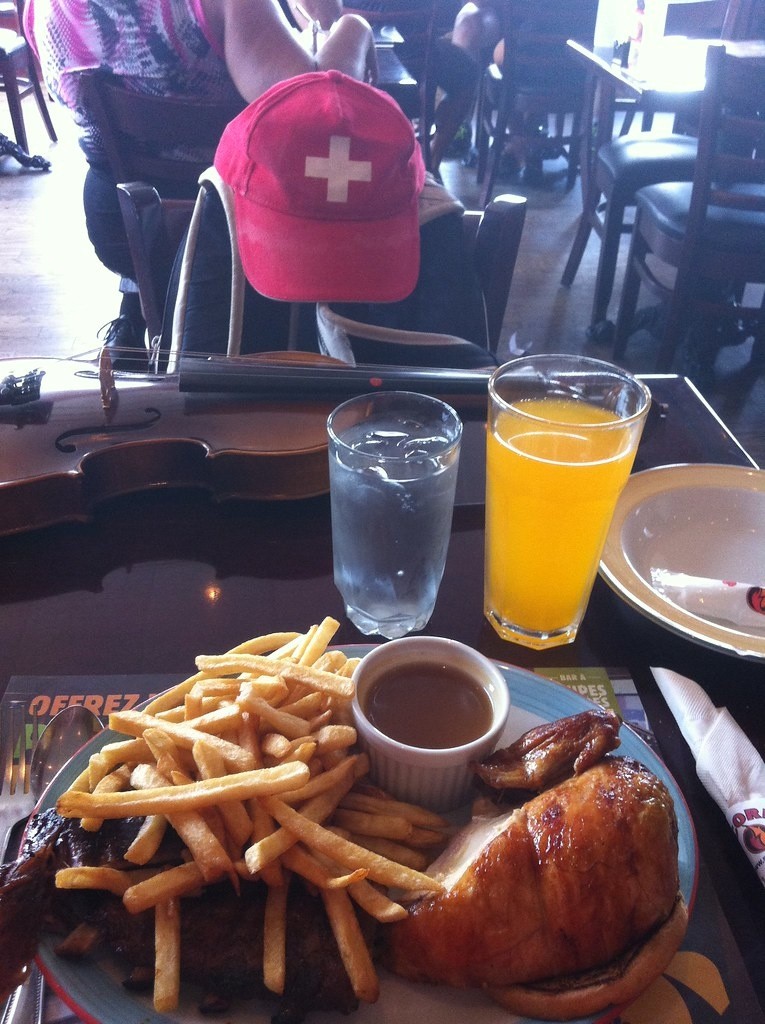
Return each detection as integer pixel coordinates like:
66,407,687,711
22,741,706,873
598,463,765,665
19,644,697,1024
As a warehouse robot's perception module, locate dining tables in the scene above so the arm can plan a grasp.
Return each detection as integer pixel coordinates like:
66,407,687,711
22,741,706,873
0,374,765,1024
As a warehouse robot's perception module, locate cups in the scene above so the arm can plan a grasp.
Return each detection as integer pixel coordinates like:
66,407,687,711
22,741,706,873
352,636,511,813
486,352,654,650
328,390,463,641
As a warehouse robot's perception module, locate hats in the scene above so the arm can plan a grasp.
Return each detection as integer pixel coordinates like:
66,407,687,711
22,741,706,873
212,70,426,302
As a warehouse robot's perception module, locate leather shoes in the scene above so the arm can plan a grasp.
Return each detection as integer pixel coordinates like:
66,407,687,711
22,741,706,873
97,314,148,373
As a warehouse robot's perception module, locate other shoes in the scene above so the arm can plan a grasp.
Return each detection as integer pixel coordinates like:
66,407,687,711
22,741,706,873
451,119,471,151
499,149,521,186
524,166,542,190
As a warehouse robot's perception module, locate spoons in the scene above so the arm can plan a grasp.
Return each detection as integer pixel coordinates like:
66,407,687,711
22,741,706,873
0,706,104,1024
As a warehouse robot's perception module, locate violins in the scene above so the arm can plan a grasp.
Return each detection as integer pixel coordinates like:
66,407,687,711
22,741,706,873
1,345,672,541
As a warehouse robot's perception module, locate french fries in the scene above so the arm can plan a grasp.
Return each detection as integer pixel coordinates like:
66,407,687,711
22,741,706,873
50,617,450,1010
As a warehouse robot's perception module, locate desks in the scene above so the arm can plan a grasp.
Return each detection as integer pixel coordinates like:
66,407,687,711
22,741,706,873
583,40,765,341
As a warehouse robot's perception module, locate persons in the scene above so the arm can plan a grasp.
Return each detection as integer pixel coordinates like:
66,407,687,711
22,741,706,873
621,0,647,83
40,1,552,372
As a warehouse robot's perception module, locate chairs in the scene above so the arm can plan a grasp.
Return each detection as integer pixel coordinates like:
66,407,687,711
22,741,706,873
561,40,718,336
614,41,765,370
467,0,589,207
116,179,529,375
0,0,59,156
341,0,440,174
